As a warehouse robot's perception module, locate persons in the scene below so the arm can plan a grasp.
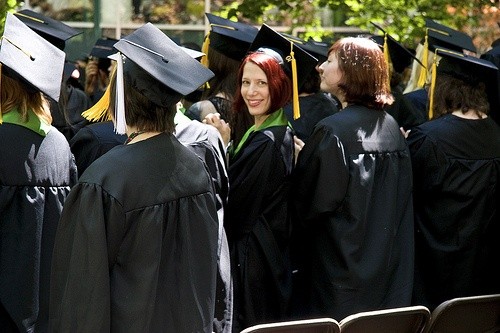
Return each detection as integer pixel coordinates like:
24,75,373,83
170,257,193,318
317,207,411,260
1,7,500,333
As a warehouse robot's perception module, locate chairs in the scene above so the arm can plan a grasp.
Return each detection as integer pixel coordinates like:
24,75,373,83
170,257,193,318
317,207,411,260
238,292,500,333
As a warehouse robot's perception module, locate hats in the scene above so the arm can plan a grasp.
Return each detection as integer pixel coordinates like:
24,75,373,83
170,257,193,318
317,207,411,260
202,10,260,62
0,11,66,106
434,42,500,87
90,36,122,64
9,8,84,52
174,40,206,62
248,22,321,93
113,22,217,109
365,20,429,74
309,36,336,49
280,32,329,62
422,17,479,60
